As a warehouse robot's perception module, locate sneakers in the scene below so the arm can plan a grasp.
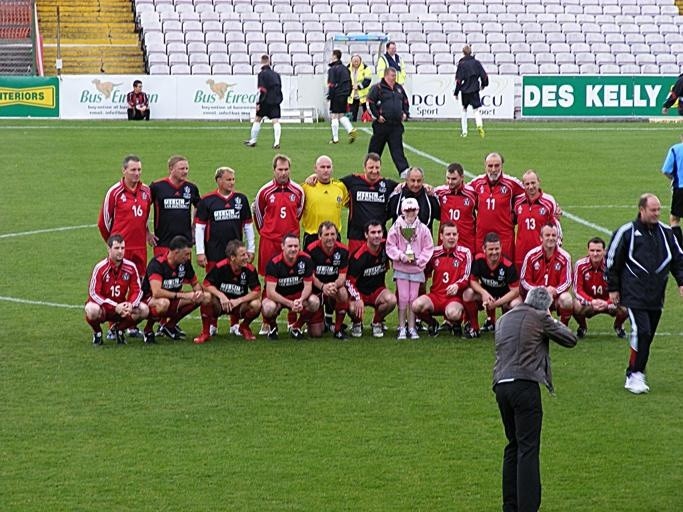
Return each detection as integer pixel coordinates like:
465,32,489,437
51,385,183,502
461,133,467,137
624,371,650,394
244,141,256,147
478,126,485,138
577,327,587,338
613,322,626,338
272,144,280,149
329,139,338,144
349,128,358,144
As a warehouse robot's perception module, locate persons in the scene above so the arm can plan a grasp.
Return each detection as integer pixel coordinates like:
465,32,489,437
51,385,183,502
346,54,374,123
572,237,630,339
377,41,407,90
604,193,683,395
491,286,579,512
242,55,283,149
324,49,357,144
453,46,489,138
661,133,683,251
126,80,151,121
662,73,683,116
365,67,412,179
82,151,575,346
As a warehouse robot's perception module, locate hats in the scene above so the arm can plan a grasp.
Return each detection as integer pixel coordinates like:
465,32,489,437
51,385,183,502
402,198,419,211
